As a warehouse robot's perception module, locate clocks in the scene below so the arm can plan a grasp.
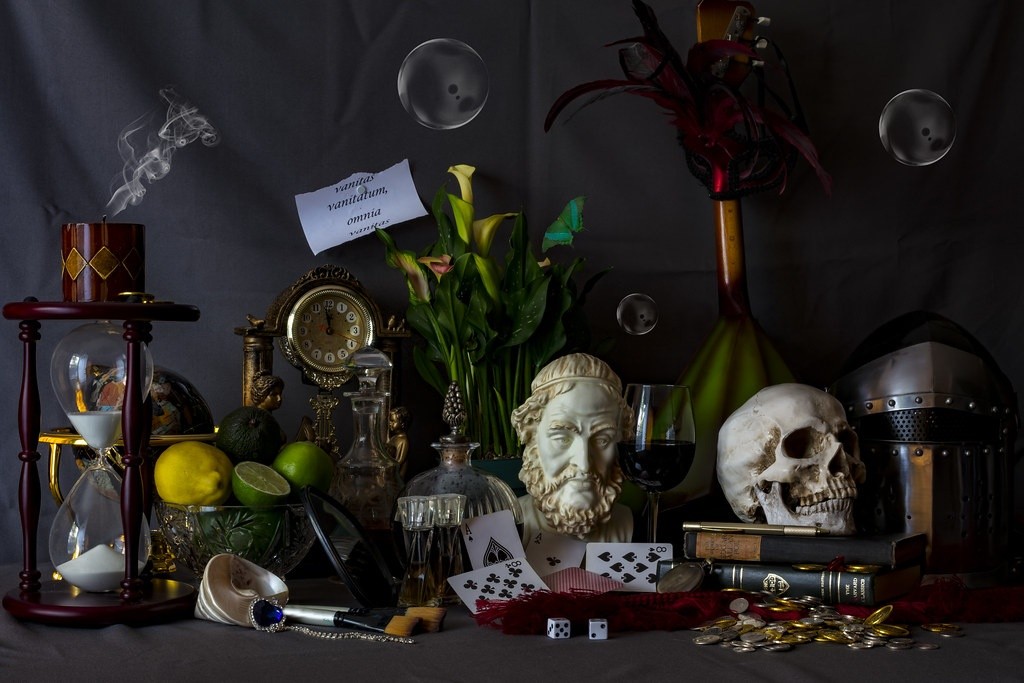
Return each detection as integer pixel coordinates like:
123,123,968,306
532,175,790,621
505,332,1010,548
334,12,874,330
288,285,375,374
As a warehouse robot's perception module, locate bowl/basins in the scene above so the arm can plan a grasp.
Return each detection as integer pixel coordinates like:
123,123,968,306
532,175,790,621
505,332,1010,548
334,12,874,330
154,500,321,581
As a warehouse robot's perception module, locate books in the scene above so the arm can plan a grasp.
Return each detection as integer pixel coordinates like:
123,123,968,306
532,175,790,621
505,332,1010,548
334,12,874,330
654,529,926,606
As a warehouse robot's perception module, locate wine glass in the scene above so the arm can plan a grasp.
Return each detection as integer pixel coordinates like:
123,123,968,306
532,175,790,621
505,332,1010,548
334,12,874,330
616,383,697,543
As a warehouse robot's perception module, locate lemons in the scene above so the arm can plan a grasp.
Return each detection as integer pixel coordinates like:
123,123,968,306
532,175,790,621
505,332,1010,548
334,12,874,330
153,440,334,506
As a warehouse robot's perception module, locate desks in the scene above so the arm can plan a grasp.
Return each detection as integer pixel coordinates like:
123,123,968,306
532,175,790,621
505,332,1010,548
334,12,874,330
2,565,1023,683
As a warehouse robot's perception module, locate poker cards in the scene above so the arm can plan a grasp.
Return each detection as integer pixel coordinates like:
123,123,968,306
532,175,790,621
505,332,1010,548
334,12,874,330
446,509,673,615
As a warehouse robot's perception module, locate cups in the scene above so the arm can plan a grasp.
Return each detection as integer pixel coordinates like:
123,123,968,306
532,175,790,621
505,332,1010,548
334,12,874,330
58,223,148,305
428,491,467,605
397,494,439,607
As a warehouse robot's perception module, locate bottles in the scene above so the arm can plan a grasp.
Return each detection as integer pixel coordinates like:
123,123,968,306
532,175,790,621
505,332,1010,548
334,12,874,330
402,381,526,549
329,350,408,546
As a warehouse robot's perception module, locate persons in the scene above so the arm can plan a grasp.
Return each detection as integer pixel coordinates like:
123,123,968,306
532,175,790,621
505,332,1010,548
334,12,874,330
509,352,636,567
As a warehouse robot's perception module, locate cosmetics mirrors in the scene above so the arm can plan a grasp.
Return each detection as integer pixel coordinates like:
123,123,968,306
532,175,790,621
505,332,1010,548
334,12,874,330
301,483,397,610
